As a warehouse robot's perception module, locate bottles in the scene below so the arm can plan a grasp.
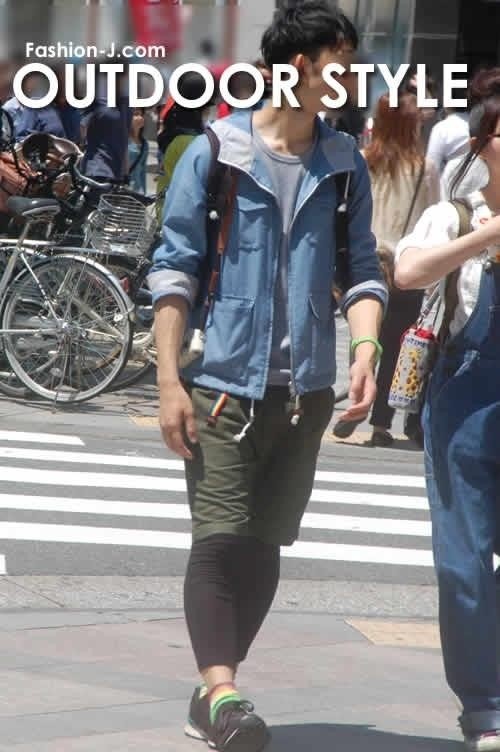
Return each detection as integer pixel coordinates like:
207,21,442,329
387,327,434,413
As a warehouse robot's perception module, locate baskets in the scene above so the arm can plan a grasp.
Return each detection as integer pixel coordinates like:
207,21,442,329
91,192,159,259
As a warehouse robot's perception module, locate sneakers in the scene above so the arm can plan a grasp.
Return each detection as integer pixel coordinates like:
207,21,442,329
334,412,368,438
184,681,270,751
371,431,395,446
462,723,500,752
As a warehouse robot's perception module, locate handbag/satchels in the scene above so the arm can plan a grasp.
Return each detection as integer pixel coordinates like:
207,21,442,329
387,283,444,415
